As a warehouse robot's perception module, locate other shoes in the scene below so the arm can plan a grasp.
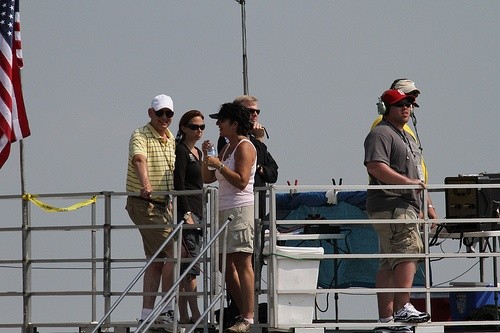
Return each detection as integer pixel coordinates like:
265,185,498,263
177,318,204,324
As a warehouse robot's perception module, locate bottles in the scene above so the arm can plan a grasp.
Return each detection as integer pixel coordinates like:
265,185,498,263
207,140,217,171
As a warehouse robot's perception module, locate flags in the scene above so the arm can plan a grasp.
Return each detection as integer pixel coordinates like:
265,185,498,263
0,0,31,170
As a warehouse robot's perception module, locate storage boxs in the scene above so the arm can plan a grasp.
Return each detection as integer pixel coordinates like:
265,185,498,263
449,281,500,320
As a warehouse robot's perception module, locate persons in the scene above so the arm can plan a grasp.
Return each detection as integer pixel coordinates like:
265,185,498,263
363,77,436,333
125,95,278,332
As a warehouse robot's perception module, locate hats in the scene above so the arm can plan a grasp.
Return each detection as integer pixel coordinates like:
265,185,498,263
151,94,173,112
381,89,415,106
209,107,248,122
390,80,420,94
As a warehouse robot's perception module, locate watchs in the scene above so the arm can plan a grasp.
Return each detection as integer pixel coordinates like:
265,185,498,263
220,163,225,172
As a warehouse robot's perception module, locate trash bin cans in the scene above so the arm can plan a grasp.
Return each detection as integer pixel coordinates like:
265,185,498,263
267,246,327,329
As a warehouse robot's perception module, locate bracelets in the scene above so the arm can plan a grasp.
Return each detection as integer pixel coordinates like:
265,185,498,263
258,164,262,171
427,205,433,207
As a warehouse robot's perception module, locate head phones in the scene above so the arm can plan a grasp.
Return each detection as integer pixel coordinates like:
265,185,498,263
376,95,389,115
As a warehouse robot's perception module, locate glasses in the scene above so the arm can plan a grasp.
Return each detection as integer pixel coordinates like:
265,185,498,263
396,102,410,107
410,92,418,97
247,108,260,115
189,124,205,130
155,110,174,118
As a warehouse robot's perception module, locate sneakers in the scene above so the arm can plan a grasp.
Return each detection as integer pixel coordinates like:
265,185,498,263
393,302,430,324
137,310,181,333
374,316,413,333
226,313,254,333
